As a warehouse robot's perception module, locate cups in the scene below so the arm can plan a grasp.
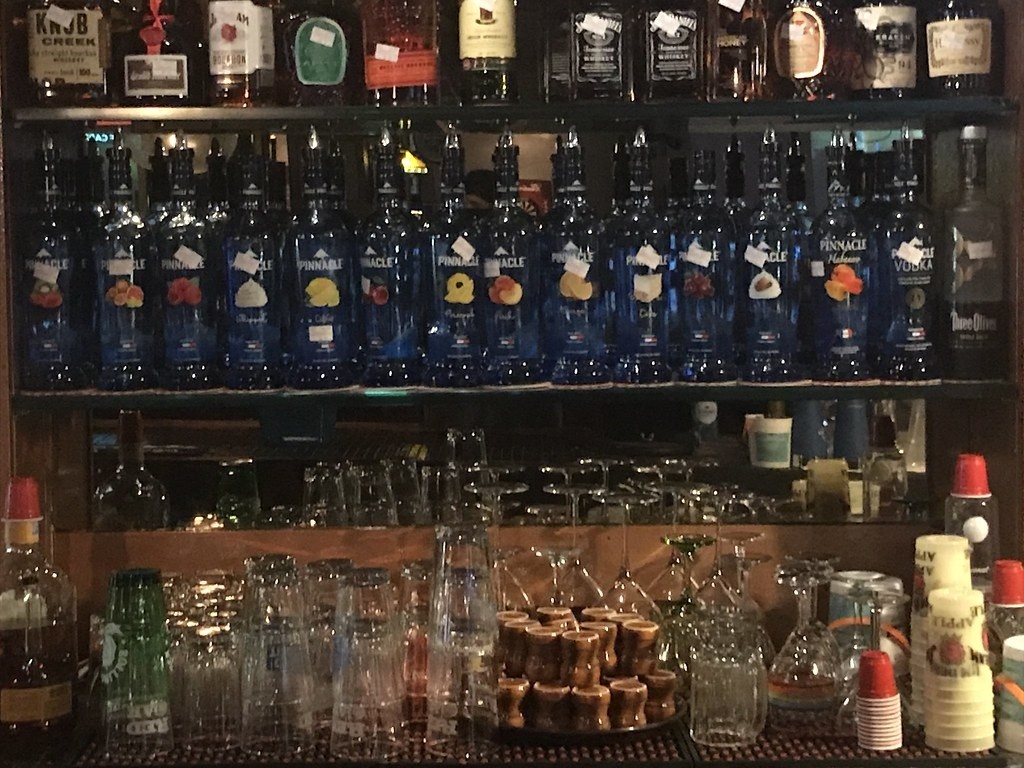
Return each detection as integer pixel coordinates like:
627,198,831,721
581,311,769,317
996,636,1024,755
912,531,994,753
857,651,904,751
689,642,769,747
98,523,680,755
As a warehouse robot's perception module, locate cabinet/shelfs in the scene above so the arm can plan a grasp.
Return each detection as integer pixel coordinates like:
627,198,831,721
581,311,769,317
0,0,1024,656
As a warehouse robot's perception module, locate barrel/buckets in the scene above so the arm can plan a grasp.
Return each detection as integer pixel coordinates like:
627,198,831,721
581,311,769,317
744,413,793,469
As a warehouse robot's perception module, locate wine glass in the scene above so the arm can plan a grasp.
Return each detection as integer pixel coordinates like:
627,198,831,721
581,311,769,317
662,533,909,739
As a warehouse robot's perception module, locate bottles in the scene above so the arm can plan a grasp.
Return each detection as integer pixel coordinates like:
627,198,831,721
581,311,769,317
0,0,1024,768
